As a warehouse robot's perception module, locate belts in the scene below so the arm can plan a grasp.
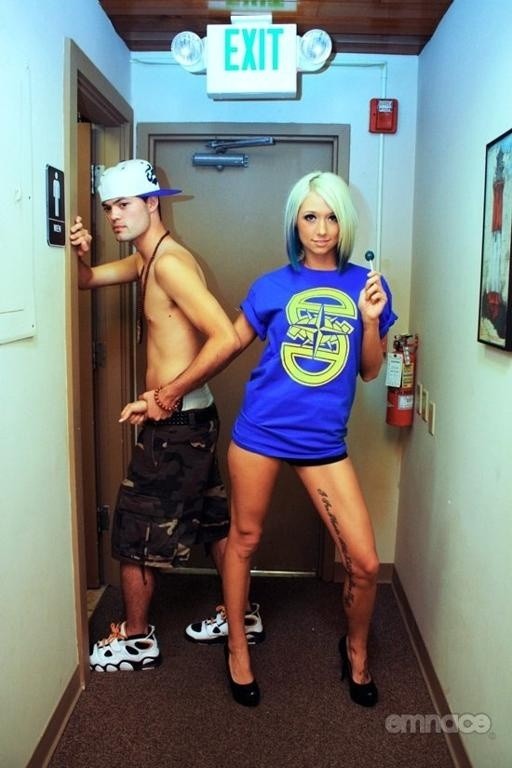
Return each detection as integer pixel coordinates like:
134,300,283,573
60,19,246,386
147,401,224,429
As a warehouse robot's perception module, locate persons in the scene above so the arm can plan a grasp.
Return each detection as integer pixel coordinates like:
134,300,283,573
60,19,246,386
116,171,398,709
69,156,268,675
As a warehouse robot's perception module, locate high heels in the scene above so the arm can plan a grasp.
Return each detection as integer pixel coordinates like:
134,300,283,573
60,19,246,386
221,631,259,711
332,632,381,706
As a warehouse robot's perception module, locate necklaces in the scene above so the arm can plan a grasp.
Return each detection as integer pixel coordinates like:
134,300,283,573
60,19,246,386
131,227,172,345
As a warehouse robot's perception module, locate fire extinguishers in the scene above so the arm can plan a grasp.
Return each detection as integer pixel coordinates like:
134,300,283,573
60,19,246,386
385,333,419,427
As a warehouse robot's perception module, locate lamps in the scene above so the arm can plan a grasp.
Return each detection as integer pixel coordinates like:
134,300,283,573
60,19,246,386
299,28,333,65
170,31,203,65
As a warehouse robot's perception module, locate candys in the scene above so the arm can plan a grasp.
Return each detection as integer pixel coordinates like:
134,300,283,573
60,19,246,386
366,251,374,272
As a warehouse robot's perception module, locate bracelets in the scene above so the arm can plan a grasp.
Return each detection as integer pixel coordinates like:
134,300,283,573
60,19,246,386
152,385,182,412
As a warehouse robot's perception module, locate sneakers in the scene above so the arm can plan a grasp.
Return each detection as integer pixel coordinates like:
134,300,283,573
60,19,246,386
85,620,162,674
182,600,270,644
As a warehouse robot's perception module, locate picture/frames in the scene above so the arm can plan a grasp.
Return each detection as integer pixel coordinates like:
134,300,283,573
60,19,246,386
477,128,512,351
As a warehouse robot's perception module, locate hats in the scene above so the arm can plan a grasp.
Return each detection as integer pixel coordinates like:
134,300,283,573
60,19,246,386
93,157,188,206
